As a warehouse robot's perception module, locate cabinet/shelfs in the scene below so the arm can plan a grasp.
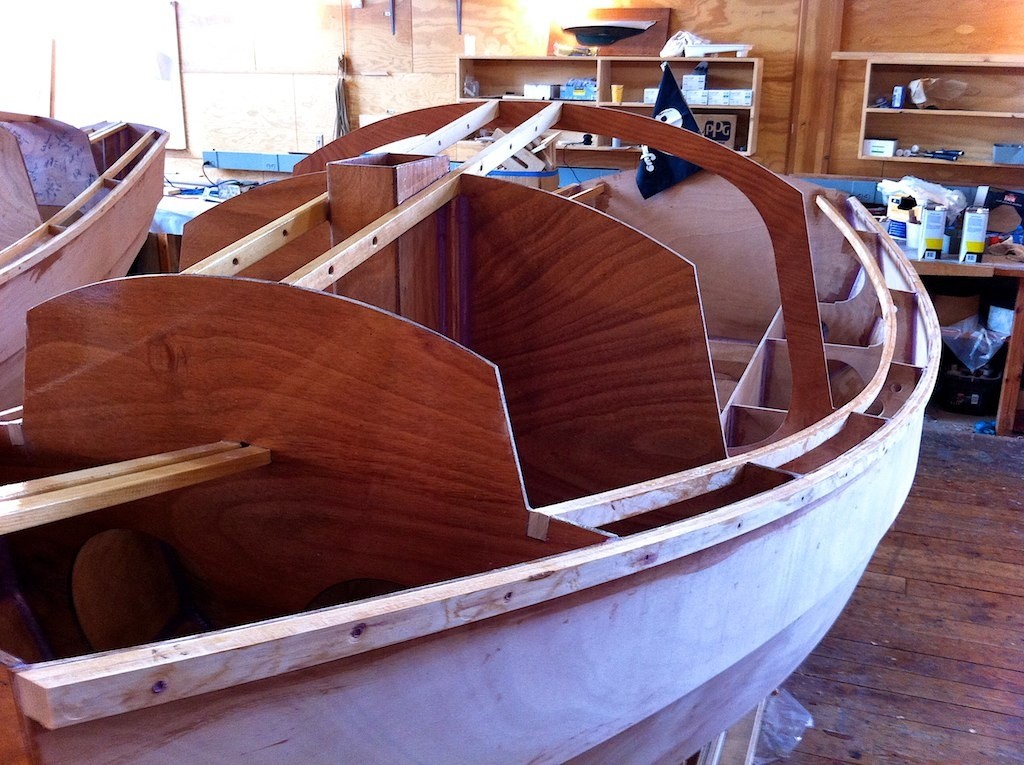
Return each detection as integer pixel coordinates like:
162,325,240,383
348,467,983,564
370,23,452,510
908,258,1024,436
857,56,1024,169
457,54,764,158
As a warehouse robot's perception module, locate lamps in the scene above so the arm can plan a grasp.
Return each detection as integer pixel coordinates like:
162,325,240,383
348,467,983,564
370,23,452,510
562,17,657,46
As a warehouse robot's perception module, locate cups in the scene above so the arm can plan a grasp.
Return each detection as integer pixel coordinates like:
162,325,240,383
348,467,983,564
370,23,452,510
610,85,624,104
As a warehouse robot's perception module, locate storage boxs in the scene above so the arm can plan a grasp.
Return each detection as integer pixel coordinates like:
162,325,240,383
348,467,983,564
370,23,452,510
523,83,560,98
931,291,983,328
558,86,597,101
891,84,905,107
993,143,1024,166
692,113,737,150
863,138,899,157
644,74,753,107
986,304,1017,333
791,171,1024,265
487,170,560,191
933,351,1001,417
